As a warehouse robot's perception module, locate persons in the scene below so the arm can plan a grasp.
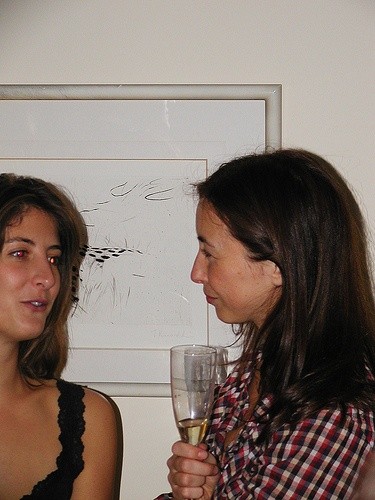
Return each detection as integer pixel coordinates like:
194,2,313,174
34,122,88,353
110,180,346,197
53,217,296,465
0,172,118,500
153,145,375,500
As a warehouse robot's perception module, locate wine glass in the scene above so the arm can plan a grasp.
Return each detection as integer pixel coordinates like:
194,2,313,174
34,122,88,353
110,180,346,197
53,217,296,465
170,345,218,500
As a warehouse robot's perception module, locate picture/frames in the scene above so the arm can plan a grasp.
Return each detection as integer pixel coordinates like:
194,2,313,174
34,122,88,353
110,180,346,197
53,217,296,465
0,83,282,397
